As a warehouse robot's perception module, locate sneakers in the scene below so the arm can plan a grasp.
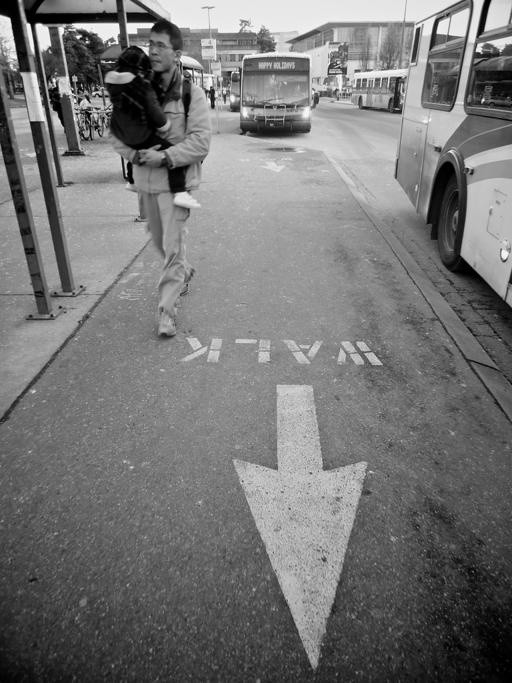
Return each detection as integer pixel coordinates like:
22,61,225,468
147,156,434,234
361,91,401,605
173,191,202,211
178,267,196,296
156,309,177,338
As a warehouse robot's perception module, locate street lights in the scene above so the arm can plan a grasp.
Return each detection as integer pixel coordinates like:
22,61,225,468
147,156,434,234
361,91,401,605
201,6,215,38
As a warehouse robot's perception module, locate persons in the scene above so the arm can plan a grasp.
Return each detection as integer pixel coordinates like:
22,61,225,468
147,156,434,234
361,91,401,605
48,75,65,128
104,21,211,337
80,91,92,110
209,86,215,109
222,86,226,104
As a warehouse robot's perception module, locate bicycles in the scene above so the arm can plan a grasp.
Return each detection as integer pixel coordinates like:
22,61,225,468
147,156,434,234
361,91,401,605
79,108,104,140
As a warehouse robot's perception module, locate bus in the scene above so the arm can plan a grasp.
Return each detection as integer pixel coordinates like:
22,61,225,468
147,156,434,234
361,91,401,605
351,69,409,113
230,51,311,134
394,0,512,308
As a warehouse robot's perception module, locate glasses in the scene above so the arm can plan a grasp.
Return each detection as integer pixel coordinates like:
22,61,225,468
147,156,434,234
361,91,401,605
147,43,173,50
